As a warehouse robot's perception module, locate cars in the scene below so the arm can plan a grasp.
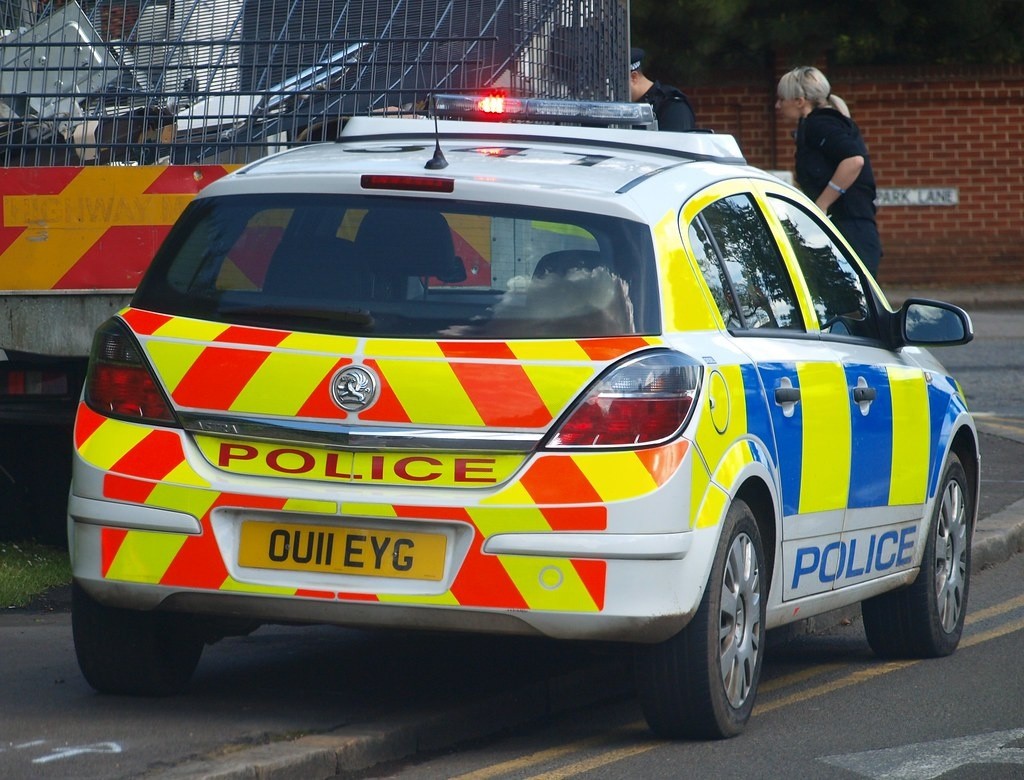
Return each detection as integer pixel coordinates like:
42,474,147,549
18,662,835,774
63,92,982,742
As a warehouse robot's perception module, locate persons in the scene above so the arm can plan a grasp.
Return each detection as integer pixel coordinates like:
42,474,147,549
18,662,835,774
628,50,697,133
775,68,884,283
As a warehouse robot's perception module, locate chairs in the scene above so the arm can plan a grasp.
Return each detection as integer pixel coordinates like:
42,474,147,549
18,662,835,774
527,248,604,279
262,237,368,305
